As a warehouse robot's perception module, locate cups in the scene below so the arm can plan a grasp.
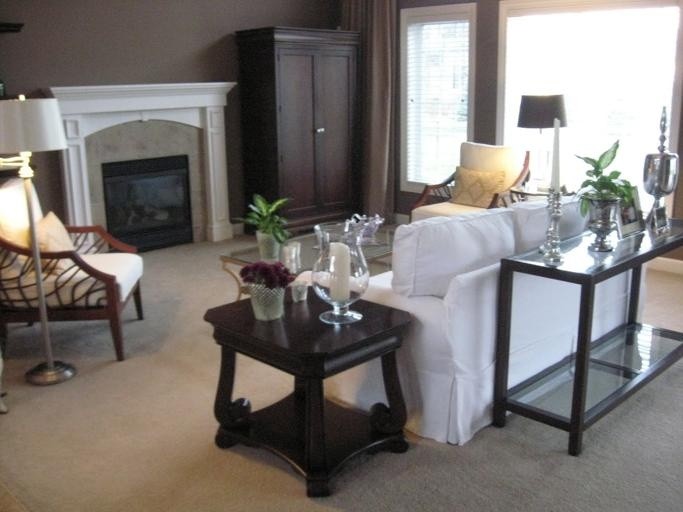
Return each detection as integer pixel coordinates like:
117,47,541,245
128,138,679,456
294,256,302,268
288,242,301,255
291,281,308,302
291,303,310,321
283,245,295,259
283,261,296,274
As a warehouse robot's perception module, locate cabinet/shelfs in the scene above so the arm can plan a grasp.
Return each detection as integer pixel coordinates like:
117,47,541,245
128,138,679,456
235,25,363,239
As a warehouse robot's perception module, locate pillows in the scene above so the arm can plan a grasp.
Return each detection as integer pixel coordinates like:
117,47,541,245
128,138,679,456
449,166,506,209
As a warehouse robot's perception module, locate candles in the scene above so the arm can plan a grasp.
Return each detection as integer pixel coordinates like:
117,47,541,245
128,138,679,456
328,240,352,302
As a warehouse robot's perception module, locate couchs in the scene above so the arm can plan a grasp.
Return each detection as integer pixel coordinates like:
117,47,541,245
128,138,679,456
0,175,144,361
409,142,530,224
324,194,648,445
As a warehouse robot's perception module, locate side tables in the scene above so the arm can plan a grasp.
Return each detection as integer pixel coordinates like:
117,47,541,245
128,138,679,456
509,186,575,204
203,284,412,497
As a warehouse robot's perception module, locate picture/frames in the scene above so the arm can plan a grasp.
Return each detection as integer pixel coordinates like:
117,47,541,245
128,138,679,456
614,186,646,240
653,207,667,234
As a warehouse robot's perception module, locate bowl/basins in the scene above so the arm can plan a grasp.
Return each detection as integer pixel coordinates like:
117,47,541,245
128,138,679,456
313,220,346,250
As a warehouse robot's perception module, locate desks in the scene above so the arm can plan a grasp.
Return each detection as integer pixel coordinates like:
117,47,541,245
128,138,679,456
492,219,683,457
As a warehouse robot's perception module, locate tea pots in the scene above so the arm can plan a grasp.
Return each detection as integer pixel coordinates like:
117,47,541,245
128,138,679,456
356,236,380,245
351,213,379,237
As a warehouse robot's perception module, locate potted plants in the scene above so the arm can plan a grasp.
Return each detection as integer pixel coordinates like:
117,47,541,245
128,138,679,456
231,192,295,258
575,139,637,252
238,261,298,321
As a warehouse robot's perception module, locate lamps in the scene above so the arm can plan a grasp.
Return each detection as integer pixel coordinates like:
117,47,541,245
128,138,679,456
0,93,77,386
517,94,568,134
